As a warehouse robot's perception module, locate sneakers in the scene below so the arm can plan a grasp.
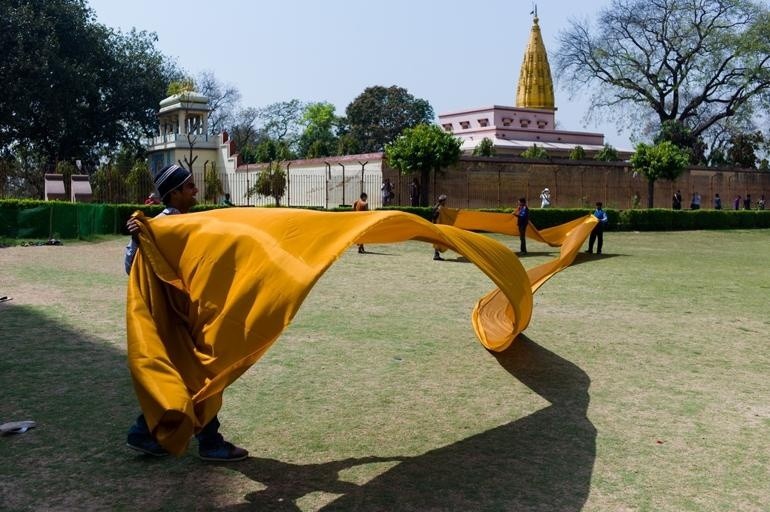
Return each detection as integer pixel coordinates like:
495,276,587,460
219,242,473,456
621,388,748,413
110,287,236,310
124,430,174,459
433,255,445,260
584,250,602,257
517,251,528,256
198,440,250,462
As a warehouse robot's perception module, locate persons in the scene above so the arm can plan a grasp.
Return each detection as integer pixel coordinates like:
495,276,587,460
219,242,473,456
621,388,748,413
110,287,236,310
539,188,552,208
691,192,702,210
631,191,641,209
408,178,420,207
432,194,459,260
743,194,754,210
352,193,369,253
756,195,765,210
759,196,766,210
125,164,250,462
512,198,530,256
381,180,394,206
585,202,608,256
733,195,742,210
672,190,682,210
221,193,235,207
145,193,160,205
714,194,723,210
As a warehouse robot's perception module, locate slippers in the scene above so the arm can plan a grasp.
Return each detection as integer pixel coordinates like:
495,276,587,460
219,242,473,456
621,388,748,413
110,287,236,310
0,420,37,435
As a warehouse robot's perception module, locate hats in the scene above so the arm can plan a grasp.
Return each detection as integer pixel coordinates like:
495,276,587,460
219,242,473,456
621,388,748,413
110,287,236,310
152,163,193,202
541,187,551,193
